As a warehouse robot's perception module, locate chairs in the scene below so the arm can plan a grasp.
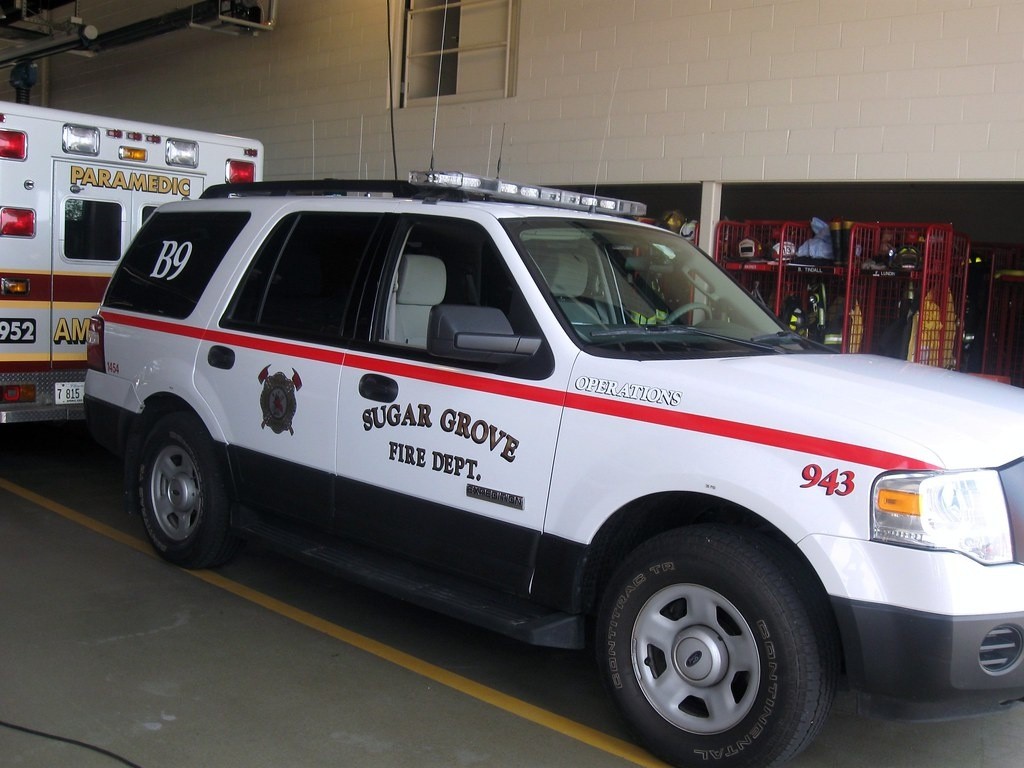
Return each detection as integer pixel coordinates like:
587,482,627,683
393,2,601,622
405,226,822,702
532,251,601,326
393,254,446,349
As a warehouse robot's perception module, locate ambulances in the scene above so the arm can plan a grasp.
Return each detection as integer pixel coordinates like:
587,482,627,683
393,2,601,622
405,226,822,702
1,102,264,423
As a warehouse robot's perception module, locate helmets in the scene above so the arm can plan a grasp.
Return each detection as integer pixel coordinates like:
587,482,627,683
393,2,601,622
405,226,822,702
662,209,685,233
679,219,699,244
772,242,797,262
892,245,920,273
735,237,763,260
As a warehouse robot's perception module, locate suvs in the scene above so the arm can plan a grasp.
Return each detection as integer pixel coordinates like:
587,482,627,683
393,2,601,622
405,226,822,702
81,167,1024,768
